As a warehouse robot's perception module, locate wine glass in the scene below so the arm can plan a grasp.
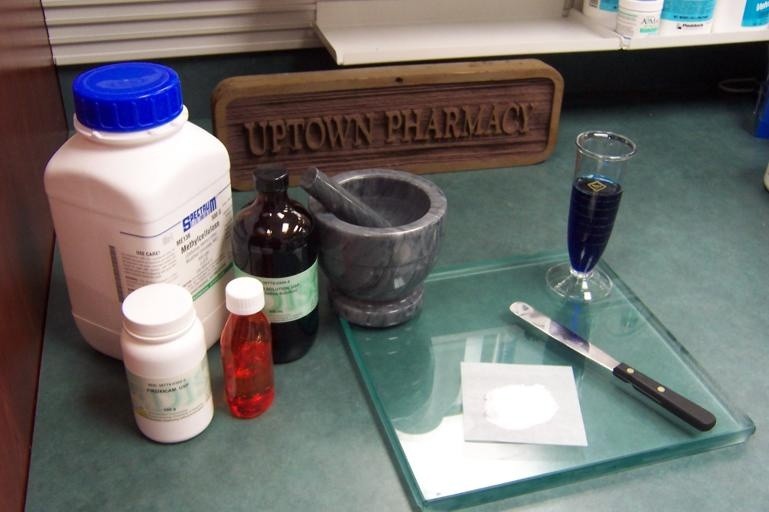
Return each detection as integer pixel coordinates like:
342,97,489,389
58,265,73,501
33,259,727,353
541,128,637,307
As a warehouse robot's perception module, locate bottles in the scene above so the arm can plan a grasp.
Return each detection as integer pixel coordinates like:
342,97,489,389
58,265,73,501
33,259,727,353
41,59,234,364
117,282,217,448
217,276,282,421
231,160,322,364
573,0,769,44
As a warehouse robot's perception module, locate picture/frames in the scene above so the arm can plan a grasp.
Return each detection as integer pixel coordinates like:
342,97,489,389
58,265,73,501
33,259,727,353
203,59,568,191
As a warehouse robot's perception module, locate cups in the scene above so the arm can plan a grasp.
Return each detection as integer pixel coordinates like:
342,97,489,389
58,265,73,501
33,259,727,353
307,167,451,331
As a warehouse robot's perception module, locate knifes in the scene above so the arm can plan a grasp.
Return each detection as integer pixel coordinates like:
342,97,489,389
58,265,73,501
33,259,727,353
511,299,717,433
